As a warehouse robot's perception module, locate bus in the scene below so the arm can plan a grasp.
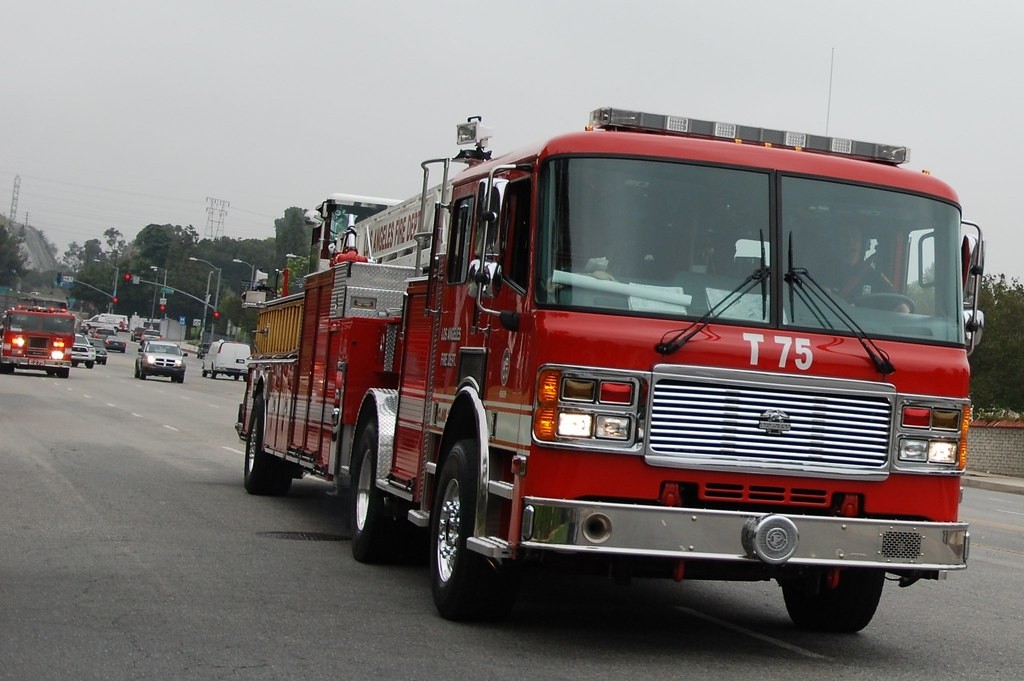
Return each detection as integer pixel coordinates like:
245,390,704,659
0,287,68,309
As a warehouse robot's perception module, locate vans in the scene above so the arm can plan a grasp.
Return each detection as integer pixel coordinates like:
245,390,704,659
80,314,129,332
201,341,251,383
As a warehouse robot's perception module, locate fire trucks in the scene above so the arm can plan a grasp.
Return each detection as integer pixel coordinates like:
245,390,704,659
234,116,983,636
0,305,78,378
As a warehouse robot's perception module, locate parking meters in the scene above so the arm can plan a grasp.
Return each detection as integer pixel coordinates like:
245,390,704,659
93,258,119,314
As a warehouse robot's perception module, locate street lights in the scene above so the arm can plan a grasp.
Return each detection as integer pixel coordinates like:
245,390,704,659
188,257,221,346
233,258,255,292
285,253,311,278
151,265,168,318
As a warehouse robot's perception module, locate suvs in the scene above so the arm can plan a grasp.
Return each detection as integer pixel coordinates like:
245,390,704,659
135,341,188,384
70,332,96,369
140,330,163,346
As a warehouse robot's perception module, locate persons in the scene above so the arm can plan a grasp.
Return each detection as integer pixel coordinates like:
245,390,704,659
818,219,909,312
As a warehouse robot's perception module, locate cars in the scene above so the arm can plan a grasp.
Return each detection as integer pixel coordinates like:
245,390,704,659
130,326,146,342
106,335,126,354
90,327,117,345
88,338,108,365
197,343,211,359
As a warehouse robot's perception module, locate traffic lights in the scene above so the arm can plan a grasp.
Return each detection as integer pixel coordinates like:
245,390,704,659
159,305,167,318
113,298,118,310
124,274,132,287
213,311,220,324
